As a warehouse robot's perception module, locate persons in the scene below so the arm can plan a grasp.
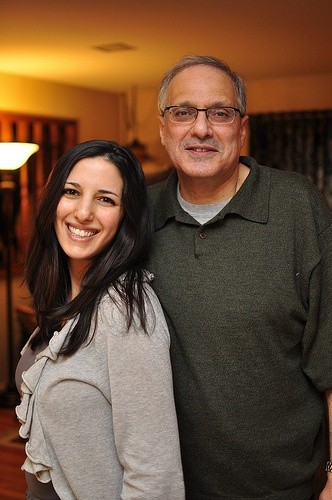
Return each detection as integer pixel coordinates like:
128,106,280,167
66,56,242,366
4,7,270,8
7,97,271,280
142,57,332,500
15,138,185,500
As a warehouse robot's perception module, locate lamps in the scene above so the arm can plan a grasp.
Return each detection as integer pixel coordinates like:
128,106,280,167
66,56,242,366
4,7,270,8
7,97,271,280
0,141,40,411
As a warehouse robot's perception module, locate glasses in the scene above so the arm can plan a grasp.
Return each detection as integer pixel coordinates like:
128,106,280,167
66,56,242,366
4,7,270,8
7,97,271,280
162,106,243,125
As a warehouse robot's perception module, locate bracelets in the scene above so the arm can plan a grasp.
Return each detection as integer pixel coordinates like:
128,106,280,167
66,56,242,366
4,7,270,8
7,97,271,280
325,461,332,473
